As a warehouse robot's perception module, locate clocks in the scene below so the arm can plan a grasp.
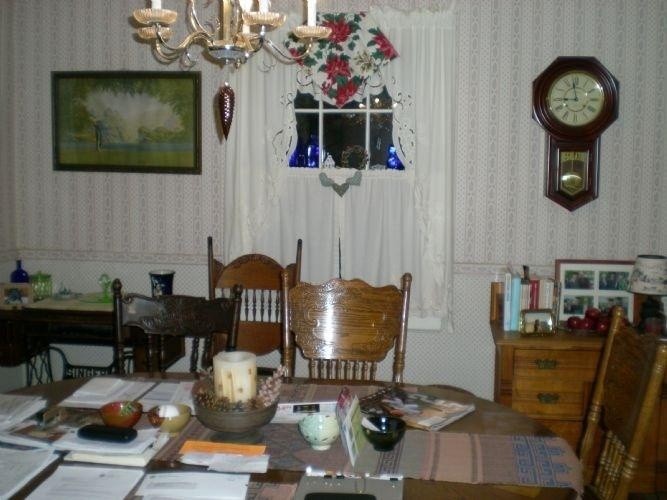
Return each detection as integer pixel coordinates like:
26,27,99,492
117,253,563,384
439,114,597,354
527,54,623,213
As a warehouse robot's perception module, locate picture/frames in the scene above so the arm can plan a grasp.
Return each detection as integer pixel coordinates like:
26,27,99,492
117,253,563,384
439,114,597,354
552,255,639,336
49,67,202,179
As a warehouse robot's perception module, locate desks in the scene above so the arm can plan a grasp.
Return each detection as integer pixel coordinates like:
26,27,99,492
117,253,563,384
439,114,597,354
1,377,585,500
0,290,147,371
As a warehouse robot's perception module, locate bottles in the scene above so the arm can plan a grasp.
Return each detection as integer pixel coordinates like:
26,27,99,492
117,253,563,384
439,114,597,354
9,260,28,284
387,137,405,168
29,270,52,302
289,134,318,166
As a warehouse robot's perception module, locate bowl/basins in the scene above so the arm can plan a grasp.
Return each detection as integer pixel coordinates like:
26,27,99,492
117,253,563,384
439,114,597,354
149,403,191,439
192,406,278,437
359,417,405,453
100,402,142,428
298,411,339,451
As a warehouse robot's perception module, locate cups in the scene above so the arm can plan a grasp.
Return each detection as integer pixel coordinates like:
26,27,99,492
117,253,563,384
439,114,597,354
214,351,257,405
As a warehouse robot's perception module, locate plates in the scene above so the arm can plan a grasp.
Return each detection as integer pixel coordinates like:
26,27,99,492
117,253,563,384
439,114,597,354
78,292,117,303
571,329,607,338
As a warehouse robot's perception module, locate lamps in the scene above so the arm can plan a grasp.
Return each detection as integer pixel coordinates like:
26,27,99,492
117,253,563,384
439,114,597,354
131,1,332,141
628,253,667,332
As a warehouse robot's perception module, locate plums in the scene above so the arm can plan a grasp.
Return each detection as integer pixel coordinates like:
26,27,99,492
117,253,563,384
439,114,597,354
568,305,628,337
643,316,663,337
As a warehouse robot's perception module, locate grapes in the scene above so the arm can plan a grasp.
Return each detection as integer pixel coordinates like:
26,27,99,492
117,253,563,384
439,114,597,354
193,388,244,413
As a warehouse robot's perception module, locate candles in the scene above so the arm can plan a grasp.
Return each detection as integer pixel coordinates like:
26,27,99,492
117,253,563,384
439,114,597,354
210,344,257,402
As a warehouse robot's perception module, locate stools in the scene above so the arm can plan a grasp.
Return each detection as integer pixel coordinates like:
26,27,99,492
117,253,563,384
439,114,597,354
0,317,56,383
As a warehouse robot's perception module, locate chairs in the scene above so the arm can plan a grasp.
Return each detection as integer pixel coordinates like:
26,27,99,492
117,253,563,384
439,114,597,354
202,231,305,376
106,276,244,376
278,267,414,385
574,302,667,500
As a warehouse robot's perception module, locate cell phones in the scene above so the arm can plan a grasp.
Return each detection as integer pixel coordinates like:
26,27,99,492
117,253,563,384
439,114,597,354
78,422,137,444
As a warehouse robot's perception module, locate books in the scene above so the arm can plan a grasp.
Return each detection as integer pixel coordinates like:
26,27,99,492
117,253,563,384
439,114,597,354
501,259,558,336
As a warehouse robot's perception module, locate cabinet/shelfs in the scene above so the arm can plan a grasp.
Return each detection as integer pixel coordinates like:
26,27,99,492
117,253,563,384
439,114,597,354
491,321,667,500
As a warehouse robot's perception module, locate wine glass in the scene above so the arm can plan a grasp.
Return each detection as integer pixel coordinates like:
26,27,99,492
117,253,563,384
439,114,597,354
100,275,111,298
